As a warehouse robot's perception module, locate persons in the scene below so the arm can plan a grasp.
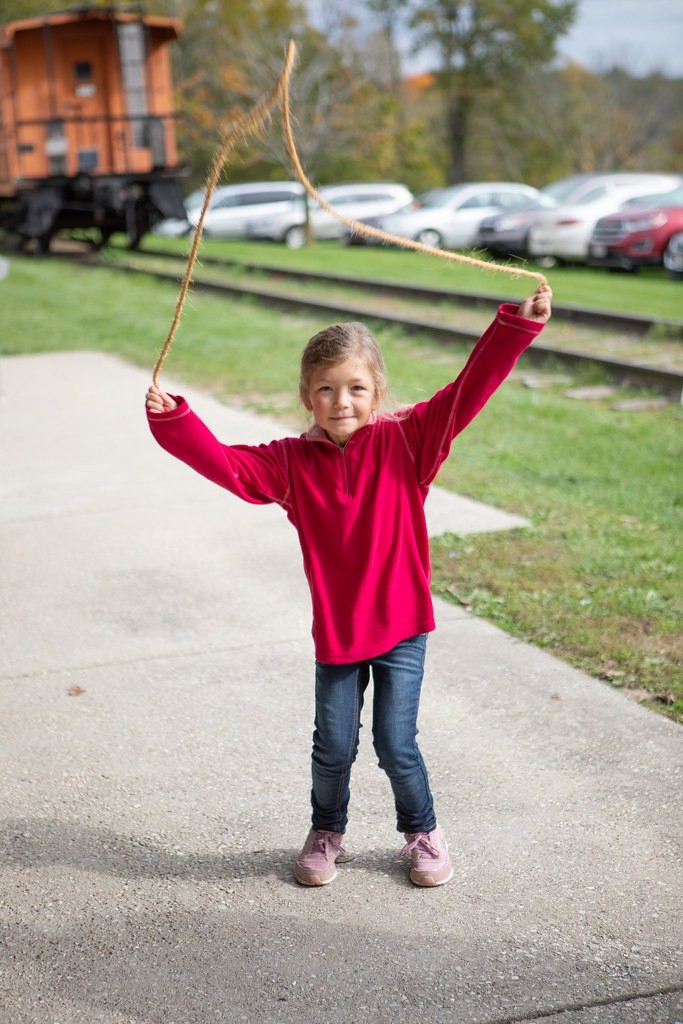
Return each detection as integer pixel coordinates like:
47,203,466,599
143,281,554,889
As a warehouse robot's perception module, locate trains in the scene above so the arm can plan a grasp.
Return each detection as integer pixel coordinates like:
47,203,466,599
0,0,190,254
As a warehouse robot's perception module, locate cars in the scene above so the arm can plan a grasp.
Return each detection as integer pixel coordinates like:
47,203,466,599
477,170,602,257
526,172,681,262
343,180,560,252
587,180,683,275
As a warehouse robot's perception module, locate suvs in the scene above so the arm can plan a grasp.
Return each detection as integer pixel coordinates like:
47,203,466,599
243,181,418,249
151,180,302,242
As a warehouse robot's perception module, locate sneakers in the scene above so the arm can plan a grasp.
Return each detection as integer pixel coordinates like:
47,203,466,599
399,824,453,887
295,828,346,885
